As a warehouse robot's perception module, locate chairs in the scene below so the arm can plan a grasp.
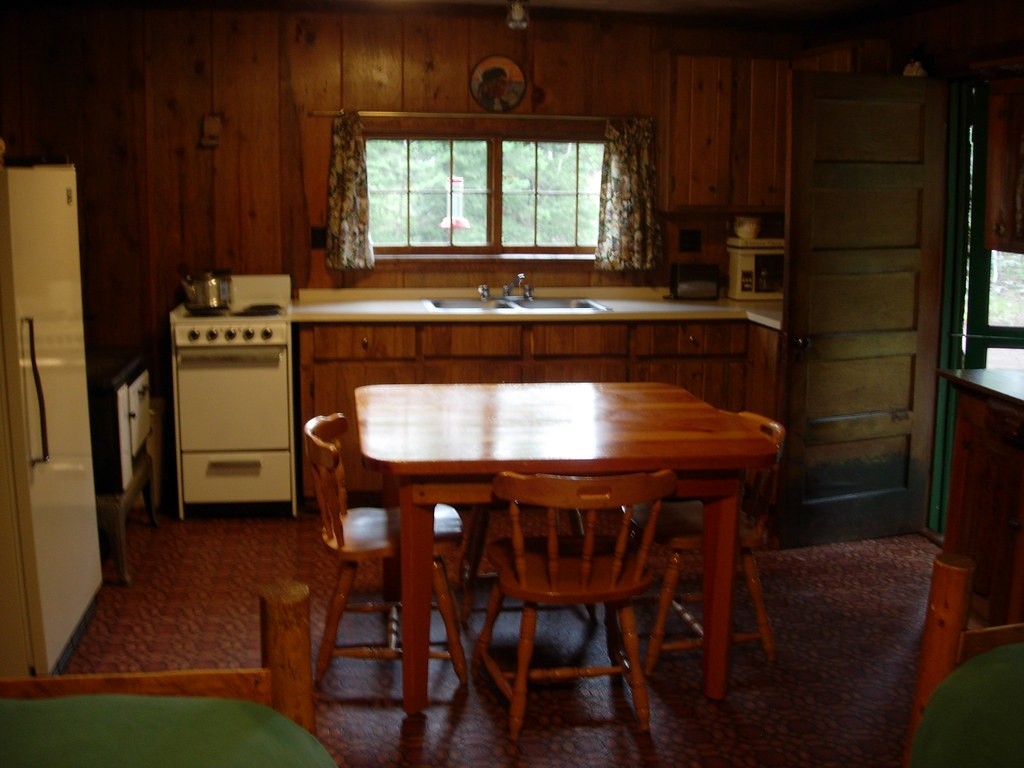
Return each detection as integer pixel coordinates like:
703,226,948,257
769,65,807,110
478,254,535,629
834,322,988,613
902,550,1024,768
303,413,470,688
629,410,786,679
0,583,339,768
471,470,678,742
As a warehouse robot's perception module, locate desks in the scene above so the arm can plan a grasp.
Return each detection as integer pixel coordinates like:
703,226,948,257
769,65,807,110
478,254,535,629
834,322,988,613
354,378,781,721
417,326,523,383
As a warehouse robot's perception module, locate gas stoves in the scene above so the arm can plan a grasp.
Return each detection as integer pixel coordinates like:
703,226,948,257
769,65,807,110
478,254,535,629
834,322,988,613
174,304,292,324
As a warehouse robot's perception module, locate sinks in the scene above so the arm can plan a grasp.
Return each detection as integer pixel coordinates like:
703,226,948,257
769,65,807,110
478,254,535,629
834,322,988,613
430,299,513,308
513,299,597,308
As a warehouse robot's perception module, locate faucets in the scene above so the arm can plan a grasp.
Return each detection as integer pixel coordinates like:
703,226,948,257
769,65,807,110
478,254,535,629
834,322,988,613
502,273,525,296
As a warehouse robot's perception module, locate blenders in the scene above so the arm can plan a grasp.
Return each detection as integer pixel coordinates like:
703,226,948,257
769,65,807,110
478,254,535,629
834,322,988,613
726,238,785,301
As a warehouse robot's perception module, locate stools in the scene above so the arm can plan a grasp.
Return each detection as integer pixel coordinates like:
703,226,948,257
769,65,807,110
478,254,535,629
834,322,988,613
96,452,161,589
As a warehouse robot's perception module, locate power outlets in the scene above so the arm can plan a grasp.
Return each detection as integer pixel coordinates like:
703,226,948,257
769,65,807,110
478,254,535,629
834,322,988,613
678,228,702,252
311,225,327,249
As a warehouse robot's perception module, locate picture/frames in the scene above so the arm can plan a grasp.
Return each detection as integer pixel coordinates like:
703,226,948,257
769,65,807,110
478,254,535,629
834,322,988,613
469,54,528,113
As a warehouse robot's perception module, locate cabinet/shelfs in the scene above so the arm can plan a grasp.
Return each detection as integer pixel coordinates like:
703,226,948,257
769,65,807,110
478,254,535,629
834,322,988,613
653,47,789,216
84,340,153,497
930,364,1024,630
628,323,749,503
520,326,628,382
298,323,420,515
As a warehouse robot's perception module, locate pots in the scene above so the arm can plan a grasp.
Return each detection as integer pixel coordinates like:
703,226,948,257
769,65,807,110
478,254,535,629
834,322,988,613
179,271,231,306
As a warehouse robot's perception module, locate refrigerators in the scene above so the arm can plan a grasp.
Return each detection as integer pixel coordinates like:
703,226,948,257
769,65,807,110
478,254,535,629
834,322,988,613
0,164,104,678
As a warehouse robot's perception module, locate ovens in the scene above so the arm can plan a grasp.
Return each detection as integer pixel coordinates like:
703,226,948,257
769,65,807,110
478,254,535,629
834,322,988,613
174,326,291,514
